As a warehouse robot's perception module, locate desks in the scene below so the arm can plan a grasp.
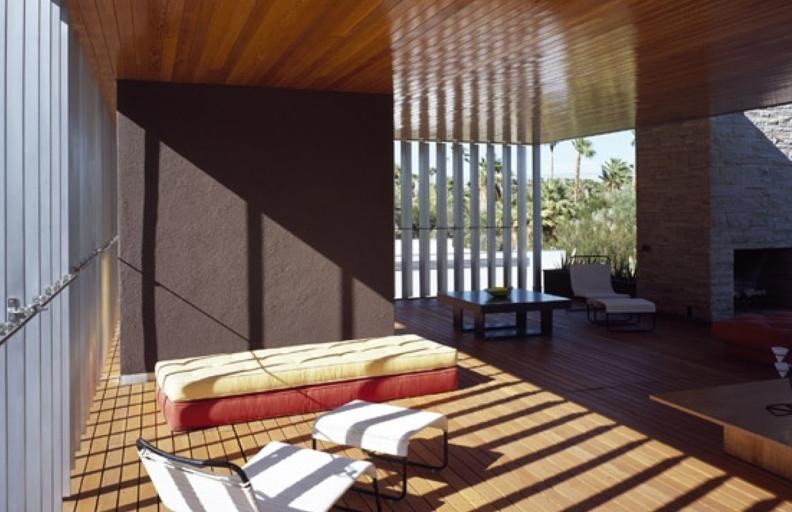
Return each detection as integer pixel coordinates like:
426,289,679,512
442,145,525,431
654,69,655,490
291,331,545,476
436,287,572,341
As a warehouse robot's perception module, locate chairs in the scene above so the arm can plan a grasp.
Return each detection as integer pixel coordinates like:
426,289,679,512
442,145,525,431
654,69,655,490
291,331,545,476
570,255,658,337
135,397,453,512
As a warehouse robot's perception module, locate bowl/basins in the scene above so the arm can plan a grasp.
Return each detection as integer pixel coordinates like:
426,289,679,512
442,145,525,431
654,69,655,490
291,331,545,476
487,288,510,296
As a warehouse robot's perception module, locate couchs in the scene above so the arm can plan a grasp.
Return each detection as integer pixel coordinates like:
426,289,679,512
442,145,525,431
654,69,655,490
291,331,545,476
714,310,792,369
